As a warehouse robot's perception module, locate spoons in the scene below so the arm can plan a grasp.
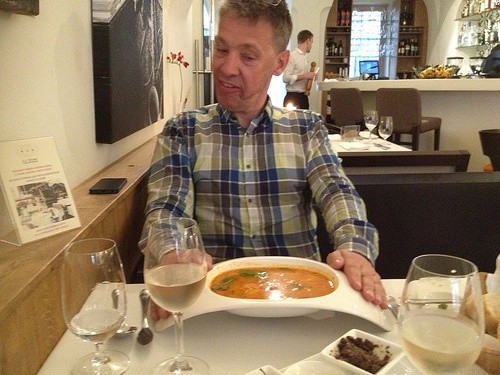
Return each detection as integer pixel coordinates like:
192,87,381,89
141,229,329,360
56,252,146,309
112,290,137,339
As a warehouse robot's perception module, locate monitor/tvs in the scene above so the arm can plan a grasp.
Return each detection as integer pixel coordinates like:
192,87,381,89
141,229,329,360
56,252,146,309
359,61,379,75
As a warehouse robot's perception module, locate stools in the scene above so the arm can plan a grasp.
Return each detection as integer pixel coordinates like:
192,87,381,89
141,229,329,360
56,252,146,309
376,87,442,151
329,88,378,135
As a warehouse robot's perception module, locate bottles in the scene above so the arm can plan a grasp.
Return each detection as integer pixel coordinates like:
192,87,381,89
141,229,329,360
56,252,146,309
397,38,419,55
326,36,343,56
402,5,408,25
337,8,350,26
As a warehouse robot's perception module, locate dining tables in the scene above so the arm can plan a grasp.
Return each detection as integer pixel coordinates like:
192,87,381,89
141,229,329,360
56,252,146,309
35,278,490,375
326,134,413,153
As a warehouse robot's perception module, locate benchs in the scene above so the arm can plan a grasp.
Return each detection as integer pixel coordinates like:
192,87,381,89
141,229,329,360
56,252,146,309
316,151,500,280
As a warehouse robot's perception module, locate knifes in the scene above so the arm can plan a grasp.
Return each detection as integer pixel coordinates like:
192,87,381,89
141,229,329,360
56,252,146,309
137,288,154,344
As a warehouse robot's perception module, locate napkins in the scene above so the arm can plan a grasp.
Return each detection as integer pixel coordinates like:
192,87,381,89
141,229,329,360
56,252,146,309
339,142,370,150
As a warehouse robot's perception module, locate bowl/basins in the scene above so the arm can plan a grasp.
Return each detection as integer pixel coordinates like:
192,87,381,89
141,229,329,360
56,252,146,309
154,256,395,333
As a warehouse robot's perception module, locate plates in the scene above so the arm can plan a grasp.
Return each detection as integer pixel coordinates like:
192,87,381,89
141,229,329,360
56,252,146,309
321,327,405,375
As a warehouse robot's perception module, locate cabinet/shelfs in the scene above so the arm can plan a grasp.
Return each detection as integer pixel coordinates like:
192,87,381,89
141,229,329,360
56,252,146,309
453,7,500,49
396,26,424,59
324,25,350,65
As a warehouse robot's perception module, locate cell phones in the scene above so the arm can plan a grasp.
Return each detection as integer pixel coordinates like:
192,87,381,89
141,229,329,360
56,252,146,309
89,178,127,194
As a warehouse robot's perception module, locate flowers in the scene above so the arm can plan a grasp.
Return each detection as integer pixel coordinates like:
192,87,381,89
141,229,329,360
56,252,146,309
165,51,190,103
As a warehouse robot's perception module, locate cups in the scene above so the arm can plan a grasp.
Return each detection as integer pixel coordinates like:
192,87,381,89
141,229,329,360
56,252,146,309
395,253,486,374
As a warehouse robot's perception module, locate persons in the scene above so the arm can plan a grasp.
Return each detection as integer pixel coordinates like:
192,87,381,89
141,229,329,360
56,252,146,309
284,29,317,109
140,0,389,320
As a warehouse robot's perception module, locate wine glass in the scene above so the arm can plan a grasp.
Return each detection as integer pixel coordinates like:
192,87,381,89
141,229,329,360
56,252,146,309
378,116,393,146
61,238,133,375
143,218,210,375
363,109,378,144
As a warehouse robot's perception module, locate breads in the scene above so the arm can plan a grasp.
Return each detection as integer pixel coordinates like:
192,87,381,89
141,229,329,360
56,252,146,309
464,292,500,341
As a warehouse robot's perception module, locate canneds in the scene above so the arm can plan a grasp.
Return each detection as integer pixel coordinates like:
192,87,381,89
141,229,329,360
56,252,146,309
338,67,348,76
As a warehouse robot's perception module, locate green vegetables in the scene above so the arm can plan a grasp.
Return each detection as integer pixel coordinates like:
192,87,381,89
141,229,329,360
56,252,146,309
211,269,269,289
